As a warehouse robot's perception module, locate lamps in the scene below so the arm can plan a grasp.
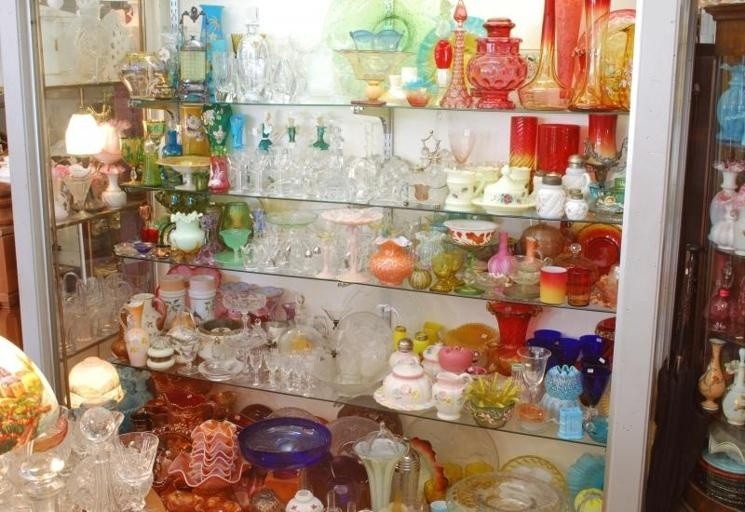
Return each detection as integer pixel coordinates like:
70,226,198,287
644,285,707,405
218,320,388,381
65,85,110,212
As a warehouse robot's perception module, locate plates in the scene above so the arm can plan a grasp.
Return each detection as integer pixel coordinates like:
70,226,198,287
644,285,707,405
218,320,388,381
1,0,694,512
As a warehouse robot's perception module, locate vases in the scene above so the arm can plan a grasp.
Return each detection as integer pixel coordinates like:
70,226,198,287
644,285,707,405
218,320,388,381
698,339,730,411
107,0,637,511
716,63,745,142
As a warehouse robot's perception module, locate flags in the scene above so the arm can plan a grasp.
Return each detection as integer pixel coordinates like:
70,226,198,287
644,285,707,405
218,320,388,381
698,339,730,411
716,63,745,142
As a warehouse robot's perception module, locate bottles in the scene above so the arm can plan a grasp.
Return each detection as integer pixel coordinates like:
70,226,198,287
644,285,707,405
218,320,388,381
696,63,745,426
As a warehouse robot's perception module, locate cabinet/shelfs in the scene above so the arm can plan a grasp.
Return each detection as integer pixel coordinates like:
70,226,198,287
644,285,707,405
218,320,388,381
696,63,745,426
1,0,694,512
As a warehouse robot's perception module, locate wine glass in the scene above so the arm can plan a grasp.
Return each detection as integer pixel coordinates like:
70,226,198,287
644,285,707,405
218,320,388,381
0,1,639,512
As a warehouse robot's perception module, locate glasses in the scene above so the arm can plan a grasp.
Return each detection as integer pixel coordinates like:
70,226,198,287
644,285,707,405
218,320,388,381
65,85,110,212
